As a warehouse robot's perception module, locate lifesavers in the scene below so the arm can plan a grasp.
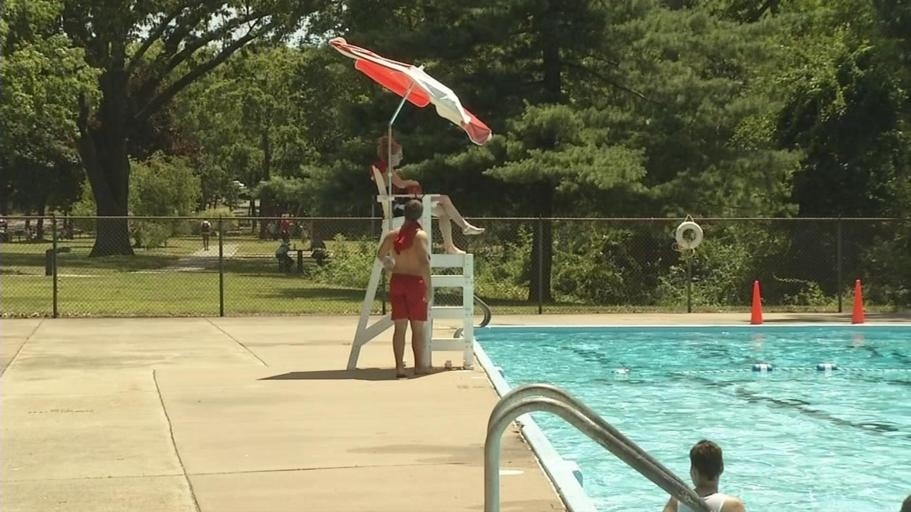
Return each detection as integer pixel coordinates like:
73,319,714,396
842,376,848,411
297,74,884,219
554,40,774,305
675,221,704,250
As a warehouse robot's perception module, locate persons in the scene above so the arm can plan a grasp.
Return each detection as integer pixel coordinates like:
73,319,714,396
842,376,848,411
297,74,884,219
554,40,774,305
199,213,328,273
370,133,485,254
375,198,439,379
662,437,746,512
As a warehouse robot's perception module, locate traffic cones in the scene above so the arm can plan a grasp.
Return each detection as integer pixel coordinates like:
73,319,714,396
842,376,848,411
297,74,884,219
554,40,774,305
750,280,766,324
852,278,866,324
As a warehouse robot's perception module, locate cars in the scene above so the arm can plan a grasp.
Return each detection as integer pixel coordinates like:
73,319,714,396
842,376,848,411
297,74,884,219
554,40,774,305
234,181,245,187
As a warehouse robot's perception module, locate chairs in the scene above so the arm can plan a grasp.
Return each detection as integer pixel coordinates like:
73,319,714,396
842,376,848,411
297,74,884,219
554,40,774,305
346,165,475,371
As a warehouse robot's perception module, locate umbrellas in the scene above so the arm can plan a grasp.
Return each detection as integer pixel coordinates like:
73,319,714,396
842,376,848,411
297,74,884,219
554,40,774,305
328,35,494,232
